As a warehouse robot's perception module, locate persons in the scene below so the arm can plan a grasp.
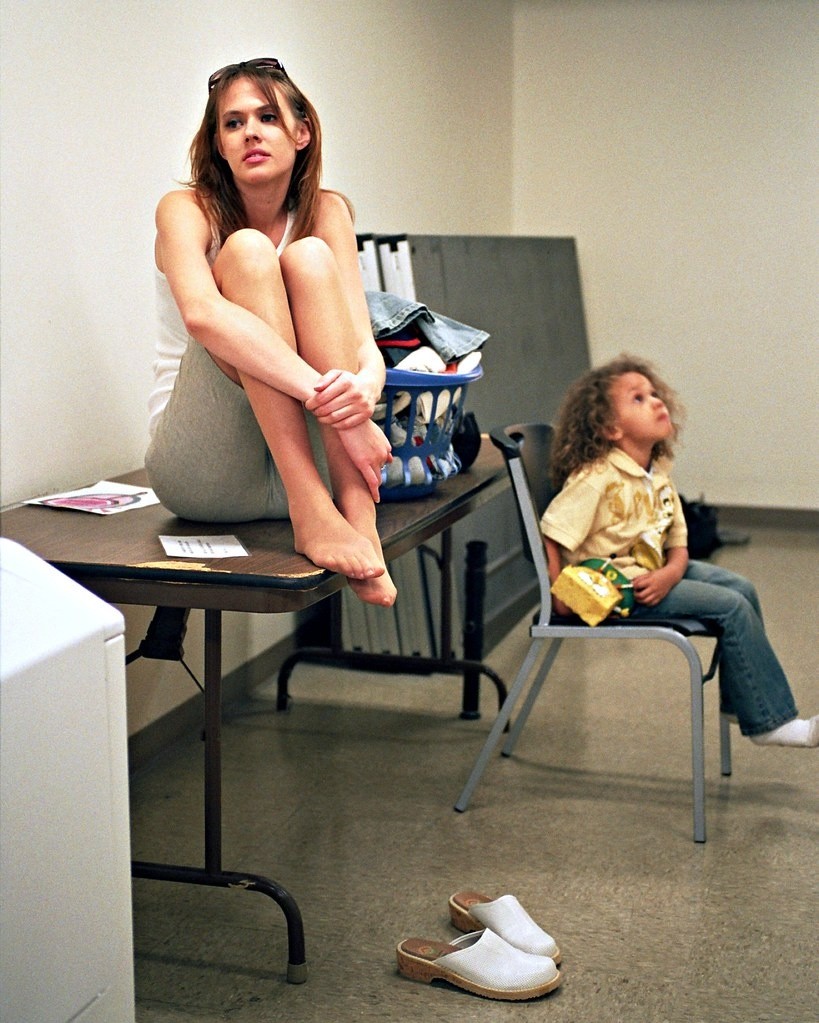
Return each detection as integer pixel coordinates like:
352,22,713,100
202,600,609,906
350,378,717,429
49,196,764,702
539,353,819,749
143,57,398,608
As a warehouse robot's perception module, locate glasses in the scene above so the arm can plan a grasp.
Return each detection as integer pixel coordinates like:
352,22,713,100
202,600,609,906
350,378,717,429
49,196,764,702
208,58,289,95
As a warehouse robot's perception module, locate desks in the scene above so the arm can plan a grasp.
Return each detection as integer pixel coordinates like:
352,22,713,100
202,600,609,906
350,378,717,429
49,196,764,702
1,419,558,985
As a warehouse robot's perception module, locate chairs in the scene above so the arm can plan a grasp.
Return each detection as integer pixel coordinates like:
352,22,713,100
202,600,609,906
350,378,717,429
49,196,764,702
455,420,732,848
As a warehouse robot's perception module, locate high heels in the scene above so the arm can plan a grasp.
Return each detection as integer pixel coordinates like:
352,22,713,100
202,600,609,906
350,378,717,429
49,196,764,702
448,890,562,965
396,927,561,1000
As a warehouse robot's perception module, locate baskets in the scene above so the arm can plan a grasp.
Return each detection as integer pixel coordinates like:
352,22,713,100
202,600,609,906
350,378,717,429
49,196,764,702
369,364,483,501
